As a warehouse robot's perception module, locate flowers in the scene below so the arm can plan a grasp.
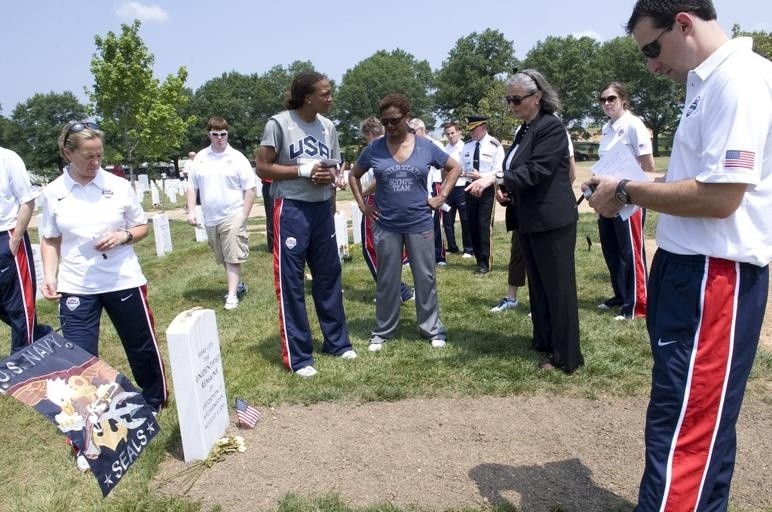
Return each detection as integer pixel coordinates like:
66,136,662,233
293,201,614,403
159,432,247,494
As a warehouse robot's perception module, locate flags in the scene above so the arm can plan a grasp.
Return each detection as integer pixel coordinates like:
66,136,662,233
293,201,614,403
235,397,262,429
723,147,757,170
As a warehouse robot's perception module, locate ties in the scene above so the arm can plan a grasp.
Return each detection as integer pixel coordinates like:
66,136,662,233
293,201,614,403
473,142,479,172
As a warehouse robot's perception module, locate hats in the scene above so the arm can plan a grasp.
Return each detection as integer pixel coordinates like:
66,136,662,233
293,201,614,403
462,117,488,131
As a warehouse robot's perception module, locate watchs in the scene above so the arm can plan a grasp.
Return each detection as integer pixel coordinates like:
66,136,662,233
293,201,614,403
615,179,631,206
121,229,134,244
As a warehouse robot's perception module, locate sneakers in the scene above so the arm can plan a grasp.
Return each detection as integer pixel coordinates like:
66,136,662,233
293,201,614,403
440,261,445,265
432,339,445,349
615,314,624,322
224,284,245,311
539,362,557,370
296,366,316,376
76,450,91,472
473,267,485,275
460,251,471,259
489,296,518,312
341,351,357,361
597,303,608,308
368,341,381,353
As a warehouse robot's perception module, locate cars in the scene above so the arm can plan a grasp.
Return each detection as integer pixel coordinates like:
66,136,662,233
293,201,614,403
572,141,600,162
104,156,189,180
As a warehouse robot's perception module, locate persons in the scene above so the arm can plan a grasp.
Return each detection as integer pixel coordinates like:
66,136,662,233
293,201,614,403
465,70,585,379
594,80,656,321
38,119,169,420
256,69,358,377
1,145,55,358
261,116,506,303
347,93,462,352
183,151,197,180
619,0,772,511
187,117,258,311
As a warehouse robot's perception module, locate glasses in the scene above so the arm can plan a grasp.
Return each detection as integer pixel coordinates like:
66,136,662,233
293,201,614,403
599,96,618,104
641,27,672,58
505,92,536,104
63,122,99,147
381,115,405,125
210,131,227,137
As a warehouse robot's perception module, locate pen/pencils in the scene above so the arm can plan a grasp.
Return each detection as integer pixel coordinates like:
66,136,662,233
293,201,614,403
574,195,584,209
